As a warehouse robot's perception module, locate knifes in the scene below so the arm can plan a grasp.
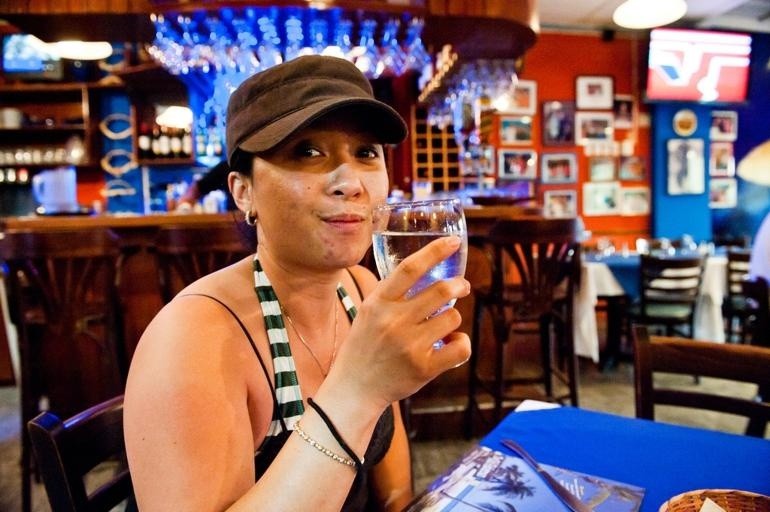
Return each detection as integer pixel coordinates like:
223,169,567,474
503,437,598,512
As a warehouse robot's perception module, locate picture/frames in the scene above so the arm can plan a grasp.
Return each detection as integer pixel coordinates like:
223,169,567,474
443,71,746,225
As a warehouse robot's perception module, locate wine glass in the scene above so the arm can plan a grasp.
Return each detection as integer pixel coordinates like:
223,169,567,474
369,199,471,370
465,143,496,193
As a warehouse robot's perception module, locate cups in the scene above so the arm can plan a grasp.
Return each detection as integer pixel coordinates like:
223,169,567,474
29,168,78,211
413,182,431,201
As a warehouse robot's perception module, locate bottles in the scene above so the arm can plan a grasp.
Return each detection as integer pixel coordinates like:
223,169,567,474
137,123,225,160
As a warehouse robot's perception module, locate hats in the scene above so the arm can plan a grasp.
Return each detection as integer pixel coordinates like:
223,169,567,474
225,53,409,168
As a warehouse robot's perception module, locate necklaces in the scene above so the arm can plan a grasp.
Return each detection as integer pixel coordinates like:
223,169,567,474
278,289,339,379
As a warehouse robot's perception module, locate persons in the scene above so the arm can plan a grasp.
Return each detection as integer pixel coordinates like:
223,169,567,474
177,161,238,210
123,55,472,512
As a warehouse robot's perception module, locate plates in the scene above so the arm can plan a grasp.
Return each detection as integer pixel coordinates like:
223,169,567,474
672,108,698,138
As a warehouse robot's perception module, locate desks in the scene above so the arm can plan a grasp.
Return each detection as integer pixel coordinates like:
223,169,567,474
578,244,754,371
0,208,580,419
403,399,770,510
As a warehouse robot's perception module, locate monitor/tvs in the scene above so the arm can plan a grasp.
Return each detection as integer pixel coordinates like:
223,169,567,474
643,25,758,110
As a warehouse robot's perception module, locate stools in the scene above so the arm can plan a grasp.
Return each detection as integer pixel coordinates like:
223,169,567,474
1,227,129,511
466,218,579,440
156,221,250,304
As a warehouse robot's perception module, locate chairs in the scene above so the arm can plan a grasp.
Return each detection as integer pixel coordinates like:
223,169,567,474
632,323,770,436
722,248,761,345
29,396,138,511
608,253,709,386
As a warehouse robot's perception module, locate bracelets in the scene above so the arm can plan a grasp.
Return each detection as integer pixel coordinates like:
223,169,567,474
293,421,366,467
307,398,364,472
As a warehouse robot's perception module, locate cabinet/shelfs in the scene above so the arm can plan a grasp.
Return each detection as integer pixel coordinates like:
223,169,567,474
0,1,429,207
